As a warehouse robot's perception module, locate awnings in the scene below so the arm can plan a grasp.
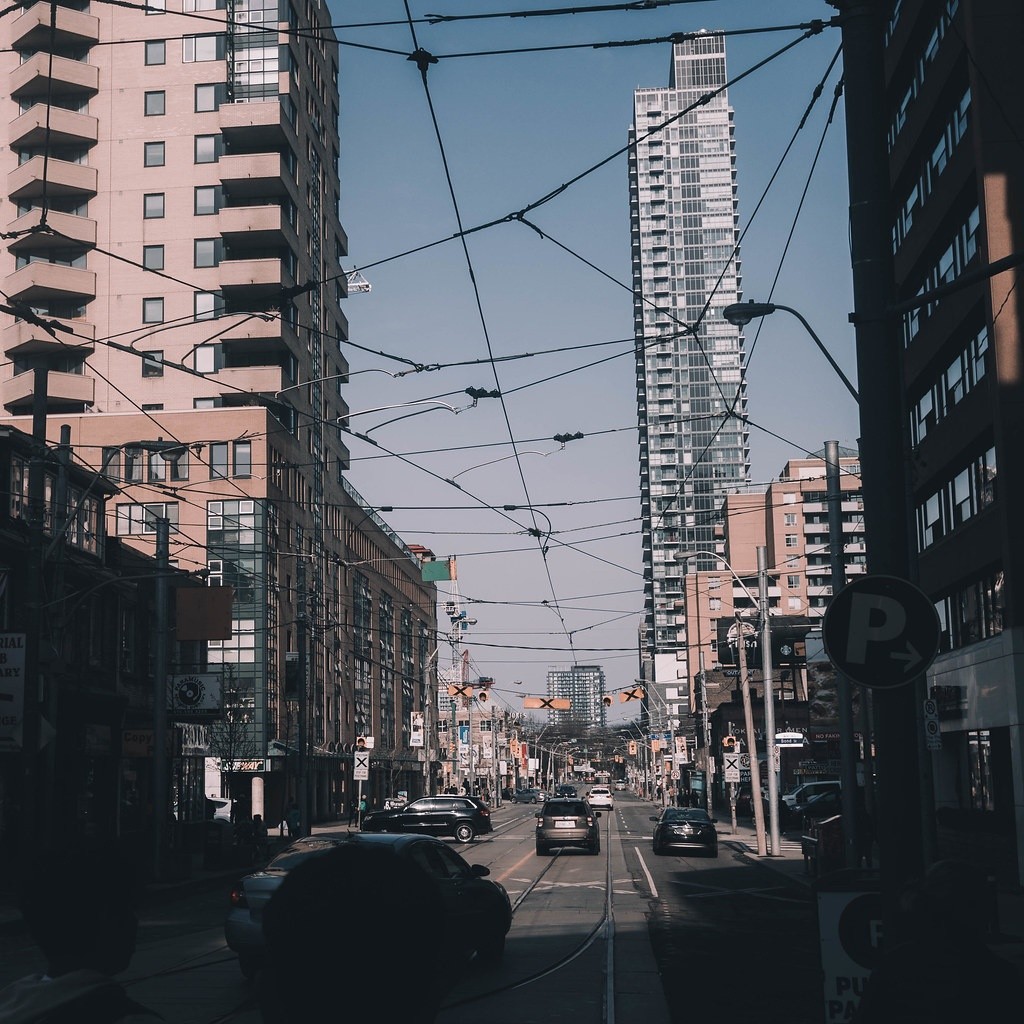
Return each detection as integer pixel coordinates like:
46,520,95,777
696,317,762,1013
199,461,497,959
574,765,588,771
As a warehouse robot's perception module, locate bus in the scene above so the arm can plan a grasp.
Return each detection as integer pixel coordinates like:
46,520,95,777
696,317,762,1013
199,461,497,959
594,769,611,791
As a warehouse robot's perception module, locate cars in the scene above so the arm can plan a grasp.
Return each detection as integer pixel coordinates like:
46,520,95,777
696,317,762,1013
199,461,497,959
584,788,615,810
226,832,511,983
509,788,552,805
650,807,719,858
613,779,626,792
788,790,843,830
729,785,765,818
501,787,523,800
554,785,578,798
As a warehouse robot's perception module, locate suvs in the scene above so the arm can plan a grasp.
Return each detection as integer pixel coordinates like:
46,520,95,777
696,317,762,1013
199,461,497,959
535,797,602,857
360,796,495,845
780,780,842,808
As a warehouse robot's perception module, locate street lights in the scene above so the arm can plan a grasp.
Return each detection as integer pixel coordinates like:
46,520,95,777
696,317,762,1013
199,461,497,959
491,680,522,808
632,682,666,807
612,716,651,804
673,545,781,858
19,439,189,940
546,738,581,793
494,693,526,806
634,678,677,809
533,724,555,789
425,617,477,797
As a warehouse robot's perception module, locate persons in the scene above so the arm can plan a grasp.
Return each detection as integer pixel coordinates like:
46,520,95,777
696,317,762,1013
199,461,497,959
212,794,215,797
750,791,789,835
506,786,512,800
0,853,169,1024
285,797,300,843
855,802,874,867
348,793,371,830
444,784,494,803
639,782,700,808
252,815,268,838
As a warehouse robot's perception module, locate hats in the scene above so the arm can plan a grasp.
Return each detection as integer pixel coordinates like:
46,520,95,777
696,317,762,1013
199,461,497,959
362,795,367,800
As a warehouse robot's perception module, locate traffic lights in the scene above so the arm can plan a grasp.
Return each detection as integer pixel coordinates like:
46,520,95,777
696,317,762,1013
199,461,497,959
723,736,736,747
476,689,490,703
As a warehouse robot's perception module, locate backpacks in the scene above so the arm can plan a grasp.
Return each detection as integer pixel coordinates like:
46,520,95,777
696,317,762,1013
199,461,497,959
359,801,366,813
